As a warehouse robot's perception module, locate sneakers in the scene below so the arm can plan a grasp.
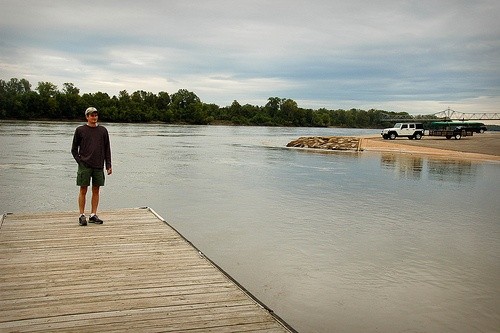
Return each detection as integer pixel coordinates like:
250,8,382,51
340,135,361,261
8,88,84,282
89,215,103,224
78,215,87,226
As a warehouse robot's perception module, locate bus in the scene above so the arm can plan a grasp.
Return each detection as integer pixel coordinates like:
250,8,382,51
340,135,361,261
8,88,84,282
430,122,489,134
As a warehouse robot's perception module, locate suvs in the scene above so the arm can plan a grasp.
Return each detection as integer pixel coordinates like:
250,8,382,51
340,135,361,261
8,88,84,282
380,122,424,140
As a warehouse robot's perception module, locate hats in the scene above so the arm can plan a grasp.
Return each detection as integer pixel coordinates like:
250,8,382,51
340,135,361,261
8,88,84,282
85,107,97,115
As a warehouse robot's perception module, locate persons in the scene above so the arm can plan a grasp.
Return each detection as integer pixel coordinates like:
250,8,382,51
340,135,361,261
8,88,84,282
70,106,113,227
